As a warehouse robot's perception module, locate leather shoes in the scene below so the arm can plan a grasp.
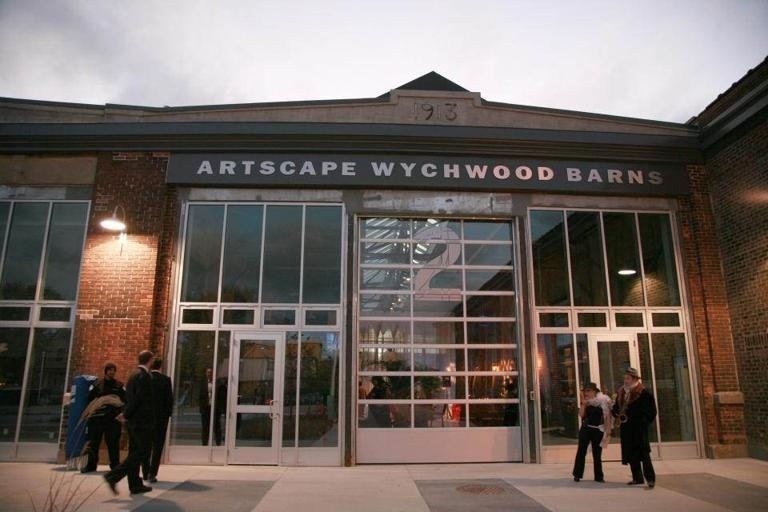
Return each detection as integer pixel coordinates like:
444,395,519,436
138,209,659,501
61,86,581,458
573,475,580,483
142,473,158,483
645,476,655,488
103,474,118,495
594,476,606,482
129,485,153,494
80,464,97,473
627,478,643,485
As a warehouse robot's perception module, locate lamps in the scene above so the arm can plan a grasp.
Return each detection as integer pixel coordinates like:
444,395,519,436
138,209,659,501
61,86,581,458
100,204,128,255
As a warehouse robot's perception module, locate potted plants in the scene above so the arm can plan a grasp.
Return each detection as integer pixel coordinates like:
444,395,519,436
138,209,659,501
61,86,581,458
388,359,442,427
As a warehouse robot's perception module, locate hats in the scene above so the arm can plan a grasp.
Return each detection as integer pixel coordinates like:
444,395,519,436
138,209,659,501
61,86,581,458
581,383,600,393
619,368,645,380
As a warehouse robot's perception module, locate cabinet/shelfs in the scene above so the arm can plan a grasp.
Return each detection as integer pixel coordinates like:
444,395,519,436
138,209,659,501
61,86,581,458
556,340,590,438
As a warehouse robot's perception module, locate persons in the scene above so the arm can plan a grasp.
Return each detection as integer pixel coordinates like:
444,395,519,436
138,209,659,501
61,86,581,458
141,357,174,482
561,383,621,443
80,363,126,473
384,348,396,372
200,367,271,446
103,349,155,493
572,382,611,483
613,368,657,487
368,376,391,426
